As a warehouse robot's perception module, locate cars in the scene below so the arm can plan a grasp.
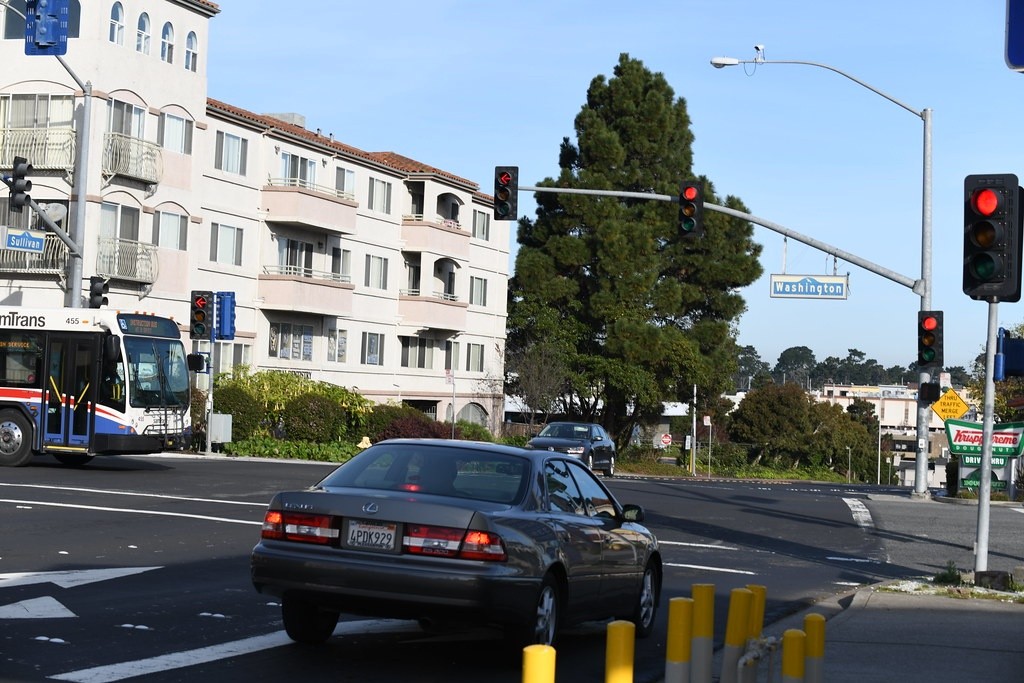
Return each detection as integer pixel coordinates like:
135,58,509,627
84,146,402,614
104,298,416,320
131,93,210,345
251,438,663,653
525,422,615,477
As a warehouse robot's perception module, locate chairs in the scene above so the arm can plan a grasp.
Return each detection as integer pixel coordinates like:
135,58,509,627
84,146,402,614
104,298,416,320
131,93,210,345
418,466,455,494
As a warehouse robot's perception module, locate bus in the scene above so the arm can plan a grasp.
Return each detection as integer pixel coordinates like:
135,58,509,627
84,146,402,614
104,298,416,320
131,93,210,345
0,307,191,466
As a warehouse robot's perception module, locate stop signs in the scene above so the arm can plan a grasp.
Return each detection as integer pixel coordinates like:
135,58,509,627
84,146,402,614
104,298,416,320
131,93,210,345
660,434,672,446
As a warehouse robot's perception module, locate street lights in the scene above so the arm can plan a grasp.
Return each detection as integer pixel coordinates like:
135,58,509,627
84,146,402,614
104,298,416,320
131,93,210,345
711,43,936,503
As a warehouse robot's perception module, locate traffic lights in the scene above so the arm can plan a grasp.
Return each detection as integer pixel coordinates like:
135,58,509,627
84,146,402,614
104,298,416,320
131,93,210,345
494,166,518,220
90,277,109,308
190,290,214,340
9,156,33,213
962,173,1024,302
918,311,943,366
678,181,704,235
